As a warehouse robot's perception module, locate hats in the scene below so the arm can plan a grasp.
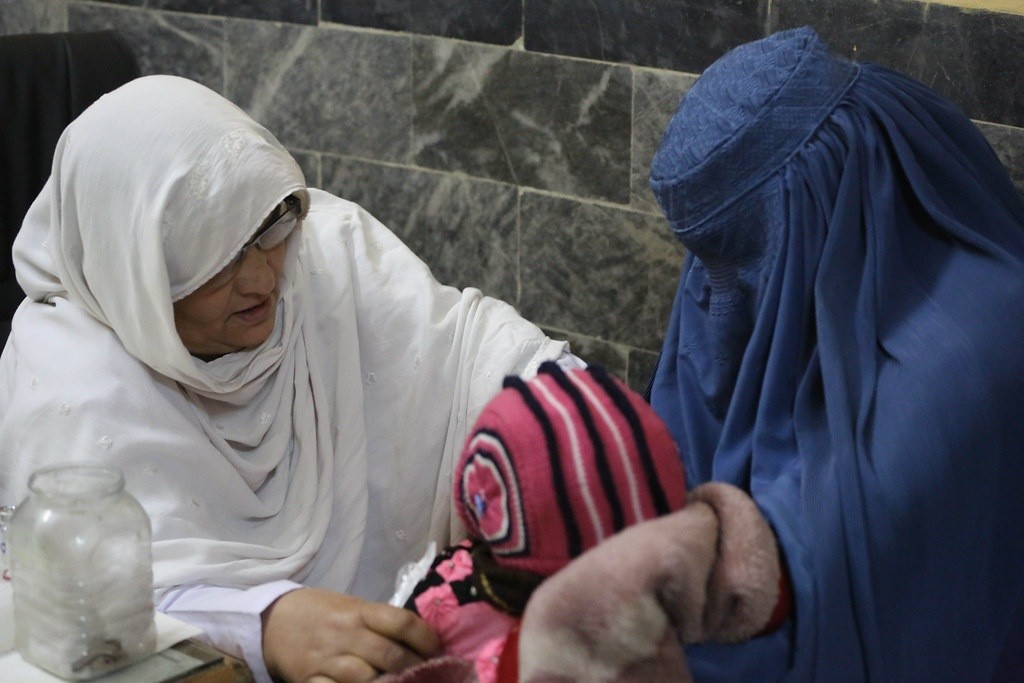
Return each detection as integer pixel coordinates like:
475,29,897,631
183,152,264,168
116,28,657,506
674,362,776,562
454,361,689,611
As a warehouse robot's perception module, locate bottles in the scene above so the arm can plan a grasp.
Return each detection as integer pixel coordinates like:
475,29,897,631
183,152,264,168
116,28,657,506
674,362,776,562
1,461,157,681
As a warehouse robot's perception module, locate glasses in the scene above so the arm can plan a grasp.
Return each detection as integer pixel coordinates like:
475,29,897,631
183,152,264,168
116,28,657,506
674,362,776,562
192,200,299,296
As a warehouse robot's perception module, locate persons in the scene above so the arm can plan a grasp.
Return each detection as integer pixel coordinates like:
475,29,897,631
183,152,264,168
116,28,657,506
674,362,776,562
390,27,1024,683
0,76,590,683
